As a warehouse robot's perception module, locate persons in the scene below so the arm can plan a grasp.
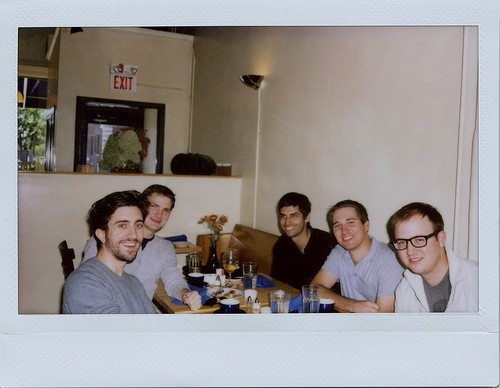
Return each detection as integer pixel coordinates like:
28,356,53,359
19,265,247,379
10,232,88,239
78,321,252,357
80,184,202,311
271,191,342,294
389,202,479,313
61,190,161,314
309,200,406,312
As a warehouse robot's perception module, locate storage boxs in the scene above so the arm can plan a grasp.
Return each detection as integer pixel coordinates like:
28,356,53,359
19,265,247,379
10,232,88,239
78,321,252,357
216,162,232,176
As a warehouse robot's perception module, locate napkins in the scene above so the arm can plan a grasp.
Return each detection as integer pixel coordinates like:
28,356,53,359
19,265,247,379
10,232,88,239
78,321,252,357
271,291,303,313
241,274,276,290
171,283,208,307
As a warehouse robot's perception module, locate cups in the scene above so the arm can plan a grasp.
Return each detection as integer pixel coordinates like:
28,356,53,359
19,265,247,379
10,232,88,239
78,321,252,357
319,298,335,313
244,290,257,302
188,273,203,288
242,261,258,290
269,289,290,313
219,299,240,314
203,274,217,283
187,252,202,272
216,268,224,280
302,285,320,313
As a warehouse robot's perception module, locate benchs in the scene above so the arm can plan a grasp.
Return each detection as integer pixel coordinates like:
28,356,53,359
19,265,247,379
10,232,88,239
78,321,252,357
229,224,280,277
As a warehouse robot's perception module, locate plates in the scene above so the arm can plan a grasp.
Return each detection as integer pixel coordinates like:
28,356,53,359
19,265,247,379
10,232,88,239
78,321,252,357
207,287,243,299
213,308,246,314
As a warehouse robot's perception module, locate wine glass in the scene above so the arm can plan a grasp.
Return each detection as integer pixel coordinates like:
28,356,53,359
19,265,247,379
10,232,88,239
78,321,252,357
222,248,238,279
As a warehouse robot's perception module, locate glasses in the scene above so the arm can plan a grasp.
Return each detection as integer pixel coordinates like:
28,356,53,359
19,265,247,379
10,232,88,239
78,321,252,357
148,203,170,215
387,230,441,250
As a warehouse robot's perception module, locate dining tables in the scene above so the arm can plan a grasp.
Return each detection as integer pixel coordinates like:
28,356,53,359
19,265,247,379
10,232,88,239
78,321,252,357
153,272,301,314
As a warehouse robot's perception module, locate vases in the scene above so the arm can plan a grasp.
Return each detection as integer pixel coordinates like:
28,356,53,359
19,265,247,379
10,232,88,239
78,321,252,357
204,243,223,274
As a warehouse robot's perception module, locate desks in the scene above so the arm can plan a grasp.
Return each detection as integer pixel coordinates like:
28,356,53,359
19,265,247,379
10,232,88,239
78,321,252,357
174,242,202,255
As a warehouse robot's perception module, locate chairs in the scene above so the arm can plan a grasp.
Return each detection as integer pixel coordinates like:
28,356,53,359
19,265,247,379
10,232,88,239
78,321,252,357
58,239,75,281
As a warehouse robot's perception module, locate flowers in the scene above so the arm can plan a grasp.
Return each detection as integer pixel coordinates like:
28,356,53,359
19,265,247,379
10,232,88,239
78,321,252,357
197,214,228,245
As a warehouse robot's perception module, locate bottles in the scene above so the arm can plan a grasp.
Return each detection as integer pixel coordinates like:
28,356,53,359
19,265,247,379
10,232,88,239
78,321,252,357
215,275,221,287
246,296,260,314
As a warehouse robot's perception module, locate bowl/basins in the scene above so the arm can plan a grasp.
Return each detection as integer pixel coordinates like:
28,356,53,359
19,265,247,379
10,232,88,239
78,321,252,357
174,241,188,248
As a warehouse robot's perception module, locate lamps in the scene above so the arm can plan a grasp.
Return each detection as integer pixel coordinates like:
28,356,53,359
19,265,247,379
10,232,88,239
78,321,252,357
239,74,264,91
